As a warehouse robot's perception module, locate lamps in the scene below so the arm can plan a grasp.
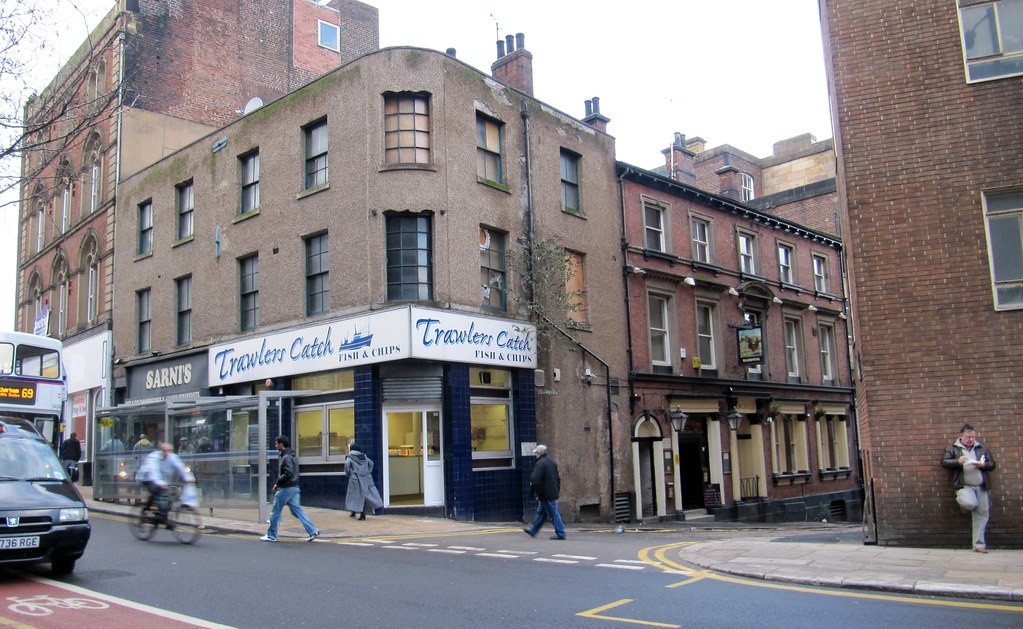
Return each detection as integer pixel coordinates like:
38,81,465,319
772,296,783,306
633,267,646,276
684,278,695,286
152,351,161,358
729,287,738,296
839,311,848,320
661,405,689,432
720,405,744,431
807,305,818,313
114,359,124,364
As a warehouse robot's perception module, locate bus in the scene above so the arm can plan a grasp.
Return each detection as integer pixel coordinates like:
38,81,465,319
0,332,68,462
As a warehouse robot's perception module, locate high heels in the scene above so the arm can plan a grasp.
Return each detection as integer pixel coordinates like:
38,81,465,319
358,512,366,520
350,512,355,517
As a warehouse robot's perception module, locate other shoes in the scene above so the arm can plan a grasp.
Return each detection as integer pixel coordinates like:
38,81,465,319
550,536,566,540
524,528,534,537
960,508,966,514
976,548,988,554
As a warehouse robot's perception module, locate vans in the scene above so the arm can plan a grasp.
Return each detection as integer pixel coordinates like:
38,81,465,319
0,415,91,577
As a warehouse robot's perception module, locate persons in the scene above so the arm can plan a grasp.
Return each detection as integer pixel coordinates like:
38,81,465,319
940,424,996,552
521,445,566,541
101,433,218,521
60,431,81,478
344,444,383,521
259,435,321,542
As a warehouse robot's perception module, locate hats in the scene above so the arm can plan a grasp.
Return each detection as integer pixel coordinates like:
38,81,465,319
533,445,548,454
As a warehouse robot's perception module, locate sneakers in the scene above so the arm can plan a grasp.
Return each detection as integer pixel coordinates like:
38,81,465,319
307,531,319,542
260,534,277,541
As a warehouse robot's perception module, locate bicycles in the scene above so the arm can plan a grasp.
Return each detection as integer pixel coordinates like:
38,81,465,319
127,482,202,545
